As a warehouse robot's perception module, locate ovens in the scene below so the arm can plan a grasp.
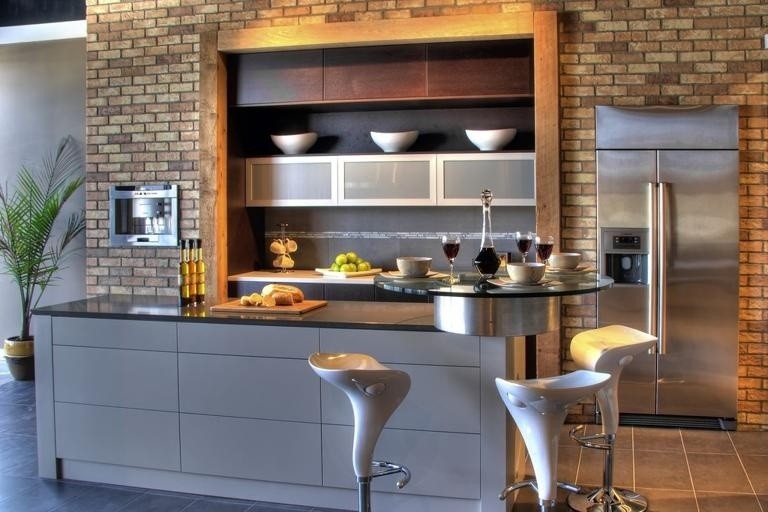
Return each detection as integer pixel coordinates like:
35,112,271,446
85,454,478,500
109,185,179,246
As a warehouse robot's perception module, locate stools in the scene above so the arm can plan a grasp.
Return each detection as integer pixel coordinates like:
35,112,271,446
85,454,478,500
493,369,611,512
563,322,661,511
307,349,413,511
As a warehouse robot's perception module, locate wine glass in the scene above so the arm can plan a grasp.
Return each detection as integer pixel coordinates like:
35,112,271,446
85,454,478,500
513,230,532,263
535,234,554,281
441,236,460,281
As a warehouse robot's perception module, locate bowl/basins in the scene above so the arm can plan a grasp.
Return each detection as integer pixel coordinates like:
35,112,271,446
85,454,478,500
268,132,318,156
369,131,420,154
548,252,581,271
396,257,432,276
464,127,518,152
507,262,546,284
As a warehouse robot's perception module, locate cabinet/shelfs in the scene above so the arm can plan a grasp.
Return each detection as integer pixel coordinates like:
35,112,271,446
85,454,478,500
427,36,535,101
324,284,428,303
230,49,325,108
229,280,323,301
324,40,427,106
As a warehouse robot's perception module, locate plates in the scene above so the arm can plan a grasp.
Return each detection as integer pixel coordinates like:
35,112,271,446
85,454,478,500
315,266,383,277
484,276,552,287
378,270,449,280
543,266,588,274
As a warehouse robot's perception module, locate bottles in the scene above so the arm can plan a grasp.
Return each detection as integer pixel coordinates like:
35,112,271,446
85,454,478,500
472,190,501,278
180,304,206,317
178,238,205,307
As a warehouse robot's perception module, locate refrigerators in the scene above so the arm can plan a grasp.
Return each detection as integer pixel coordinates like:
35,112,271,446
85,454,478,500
593,104,741,430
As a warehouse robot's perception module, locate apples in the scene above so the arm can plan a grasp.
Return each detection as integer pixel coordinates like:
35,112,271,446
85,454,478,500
330,252,371,273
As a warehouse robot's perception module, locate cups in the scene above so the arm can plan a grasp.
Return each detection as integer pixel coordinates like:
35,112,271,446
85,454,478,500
268,238,298,268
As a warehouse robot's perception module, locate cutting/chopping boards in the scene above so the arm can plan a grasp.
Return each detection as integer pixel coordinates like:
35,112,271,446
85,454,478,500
209,297,328,315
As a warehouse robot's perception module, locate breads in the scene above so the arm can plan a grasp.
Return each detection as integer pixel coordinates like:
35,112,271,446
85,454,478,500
240,284,304,307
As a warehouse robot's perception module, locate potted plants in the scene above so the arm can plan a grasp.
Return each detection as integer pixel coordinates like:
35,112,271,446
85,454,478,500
0,133,88,381
